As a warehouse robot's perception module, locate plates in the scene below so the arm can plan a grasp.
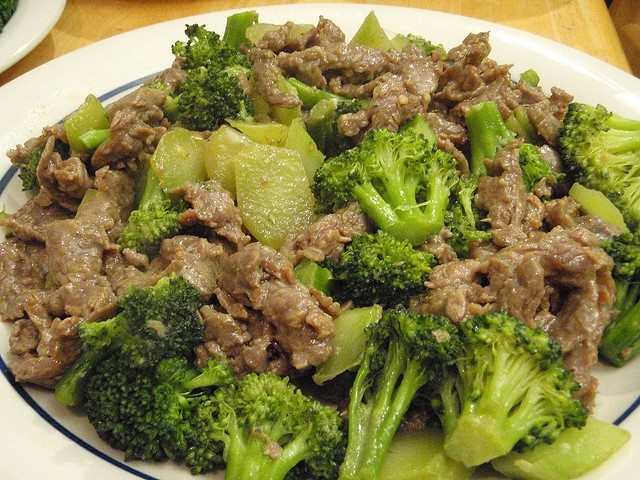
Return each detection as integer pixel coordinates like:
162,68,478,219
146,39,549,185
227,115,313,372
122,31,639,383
0,1,68,72
0,1,640,479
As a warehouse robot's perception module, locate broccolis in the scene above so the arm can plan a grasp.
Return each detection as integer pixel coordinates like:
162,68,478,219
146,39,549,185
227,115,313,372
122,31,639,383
0,10,638,479
